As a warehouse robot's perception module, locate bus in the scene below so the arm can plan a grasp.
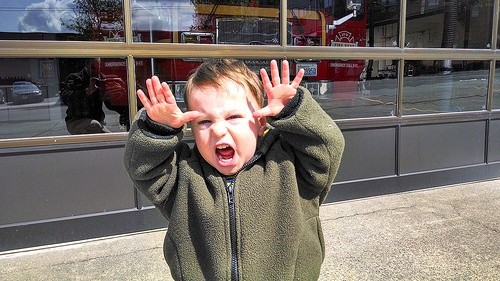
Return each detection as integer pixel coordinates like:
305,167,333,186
92,0,366,131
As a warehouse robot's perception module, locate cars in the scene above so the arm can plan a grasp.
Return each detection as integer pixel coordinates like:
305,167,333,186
10,81,44,105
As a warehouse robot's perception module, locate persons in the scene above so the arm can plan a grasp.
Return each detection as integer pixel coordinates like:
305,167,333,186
124,57,345,281
59,57,114,136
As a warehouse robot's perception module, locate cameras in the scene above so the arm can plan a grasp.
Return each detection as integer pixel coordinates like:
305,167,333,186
96,79,106,87
